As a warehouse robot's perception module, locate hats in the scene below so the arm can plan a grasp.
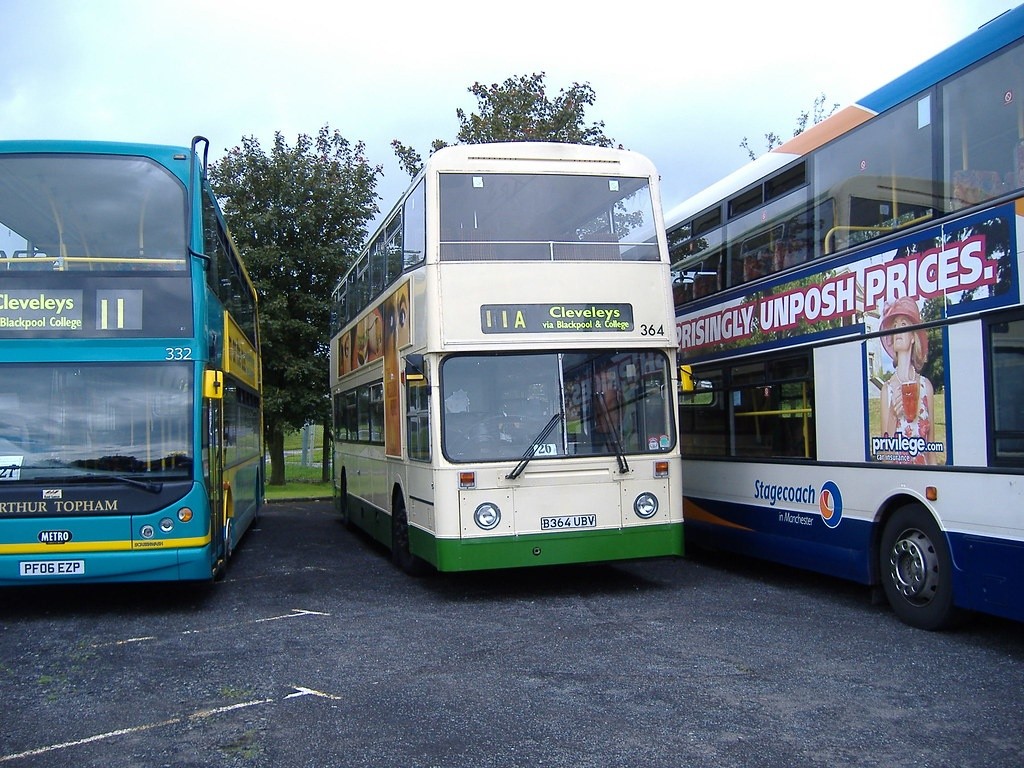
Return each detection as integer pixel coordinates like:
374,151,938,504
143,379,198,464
878,297,929,360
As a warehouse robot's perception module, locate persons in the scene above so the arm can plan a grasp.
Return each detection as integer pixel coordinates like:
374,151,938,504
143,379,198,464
591,369,627,452
880,297,934,465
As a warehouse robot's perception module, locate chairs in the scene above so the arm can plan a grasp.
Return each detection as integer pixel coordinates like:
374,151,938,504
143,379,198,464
682,406,815,458
684,237,805,305
0,247,186,271
438,216,623,260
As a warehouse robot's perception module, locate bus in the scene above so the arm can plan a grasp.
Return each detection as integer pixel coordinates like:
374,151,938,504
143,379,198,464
549,1,1024,634
1,136,268,594
328,141,696,579
